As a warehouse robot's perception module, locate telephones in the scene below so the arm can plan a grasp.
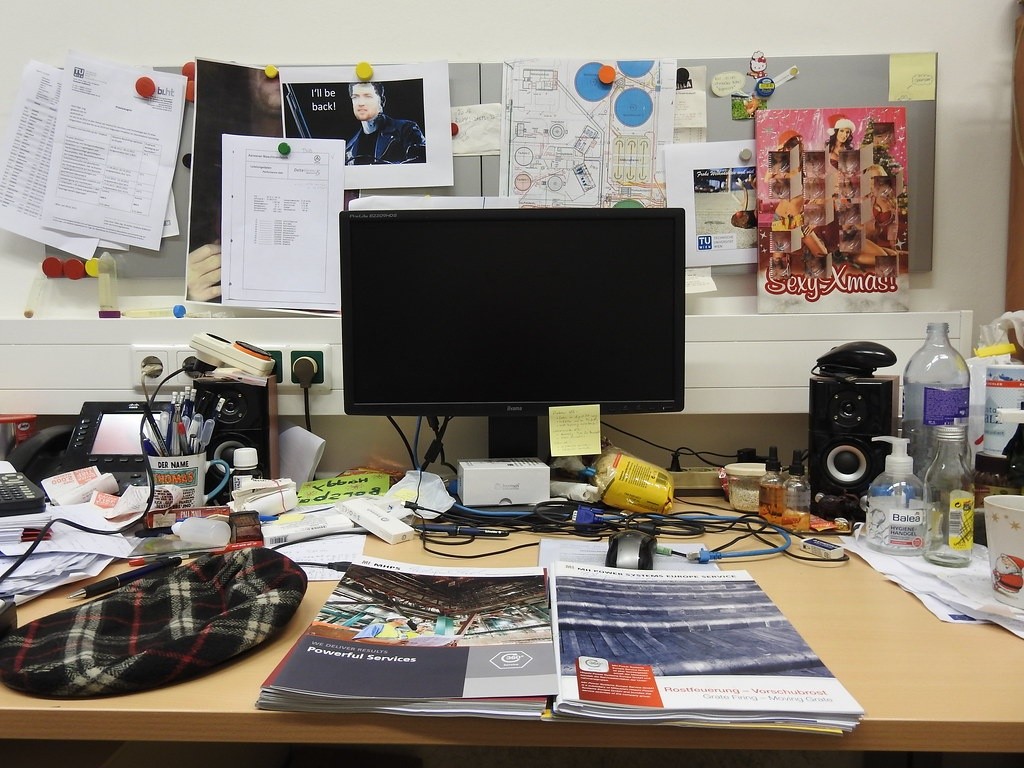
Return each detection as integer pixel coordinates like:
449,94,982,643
7,401,174,497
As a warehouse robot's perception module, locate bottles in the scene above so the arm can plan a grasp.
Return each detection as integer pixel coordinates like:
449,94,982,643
759,445,785,528
229,447,264,502
902,322,971,475
920,424,977,567
781,450,811,534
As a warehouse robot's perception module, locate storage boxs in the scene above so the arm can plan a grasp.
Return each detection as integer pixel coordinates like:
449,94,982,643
143,505,230,529
230,497,414,547
457,457,550,507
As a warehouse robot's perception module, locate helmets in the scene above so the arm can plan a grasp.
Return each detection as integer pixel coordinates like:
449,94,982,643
778,129,804,151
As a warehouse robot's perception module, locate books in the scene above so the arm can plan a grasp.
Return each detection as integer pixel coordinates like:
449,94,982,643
252,556,866,738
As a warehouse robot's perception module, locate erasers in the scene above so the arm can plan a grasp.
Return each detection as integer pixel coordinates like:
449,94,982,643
801,536,844,560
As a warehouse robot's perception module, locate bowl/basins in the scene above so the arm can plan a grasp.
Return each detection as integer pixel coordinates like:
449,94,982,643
724,462,767,513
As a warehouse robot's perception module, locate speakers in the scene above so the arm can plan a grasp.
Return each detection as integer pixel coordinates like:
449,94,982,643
809,373,900,523
194,375,280,506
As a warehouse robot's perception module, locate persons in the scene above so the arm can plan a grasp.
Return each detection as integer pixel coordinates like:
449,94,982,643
342,83,427,163
827,120,858,156
187,60,283,303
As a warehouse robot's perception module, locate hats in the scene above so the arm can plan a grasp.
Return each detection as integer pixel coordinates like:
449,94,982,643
827,113,856,135
0,546,308,699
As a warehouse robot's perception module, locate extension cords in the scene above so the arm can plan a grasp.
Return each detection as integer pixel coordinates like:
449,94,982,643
666,466,723,490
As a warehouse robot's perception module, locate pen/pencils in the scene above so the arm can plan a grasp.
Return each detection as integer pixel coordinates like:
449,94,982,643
142,387,225,457
67,556,182,599
409,523,509,537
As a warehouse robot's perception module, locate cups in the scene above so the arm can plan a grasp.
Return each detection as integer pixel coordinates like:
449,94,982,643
983,494,1024,610
145,451,231,512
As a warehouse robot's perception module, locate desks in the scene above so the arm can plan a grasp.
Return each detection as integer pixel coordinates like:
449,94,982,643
1,492,1024,767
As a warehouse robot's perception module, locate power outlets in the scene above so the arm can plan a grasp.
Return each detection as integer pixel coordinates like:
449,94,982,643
136,345,333,392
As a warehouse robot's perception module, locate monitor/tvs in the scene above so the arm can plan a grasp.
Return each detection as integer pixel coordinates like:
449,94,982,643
339,209,685,523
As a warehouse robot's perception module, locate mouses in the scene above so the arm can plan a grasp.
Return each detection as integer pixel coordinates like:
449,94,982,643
605,529,657,570
816,341,897,370
